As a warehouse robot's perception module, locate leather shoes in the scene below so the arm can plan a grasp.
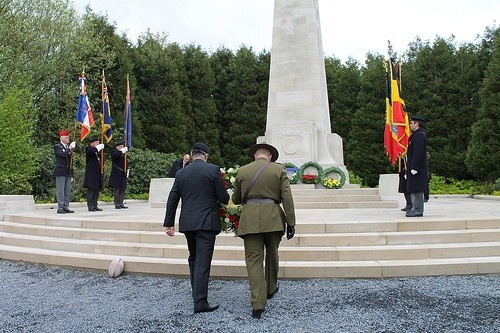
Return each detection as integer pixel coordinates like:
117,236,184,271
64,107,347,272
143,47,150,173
424,198,428,202
95,206,103,211
267,282,279,299
120,206,128,208
89,207,98,211
57,210,66,213
65,209,74,213
116,206,121,209
406,209,423,217
194,304,219,314
252,309,262,317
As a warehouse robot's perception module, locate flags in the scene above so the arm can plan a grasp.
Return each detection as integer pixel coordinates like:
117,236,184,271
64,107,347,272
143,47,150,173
384,72,392,164
100,77,113,143
388,58,412,171
76,74,95,142
124,79,132,150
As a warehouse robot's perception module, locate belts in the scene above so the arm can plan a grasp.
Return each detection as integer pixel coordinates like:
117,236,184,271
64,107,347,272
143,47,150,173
246,197,275,204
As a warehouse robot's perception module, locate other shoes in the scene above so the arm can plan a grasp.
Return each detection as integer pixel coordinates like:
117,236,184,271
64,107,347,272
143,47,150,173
401,205,412,211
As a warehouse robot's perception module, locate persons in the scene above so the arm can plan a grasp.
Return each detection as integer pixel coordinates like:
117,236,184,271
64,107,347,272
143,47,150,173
424,166,429,202
108,142,129,209
54,131,76,213
163,142,230,313
232,141,296,318
406,116,428,217
83,138,104,211
398,153,413,211
168,153,191,178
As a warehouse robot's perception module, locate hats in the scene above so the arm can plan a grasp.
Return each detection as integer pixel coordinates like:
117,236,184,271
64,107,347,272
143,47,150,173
249,144,279,162
121,147,128,153
90,137,98,142
115,142,123,145
410,116,424,121
60,131,69,136
192,142,211,154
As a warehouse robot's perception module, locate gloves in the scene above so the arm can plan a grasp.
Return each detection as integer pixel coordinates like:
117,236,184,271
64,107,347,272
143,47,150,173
287,226,295,239
404,174,407,179
127,169,129,178
70,177,73,182
95,144,104,152
411,170,417,175
70,142,76,148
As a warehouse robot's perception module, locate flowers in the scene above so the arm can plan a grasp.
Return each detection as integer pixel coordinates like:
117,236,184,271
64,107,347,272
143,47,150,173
287,172,297,181
303,174,317,181
322,177,341,188
217,164,243,235
